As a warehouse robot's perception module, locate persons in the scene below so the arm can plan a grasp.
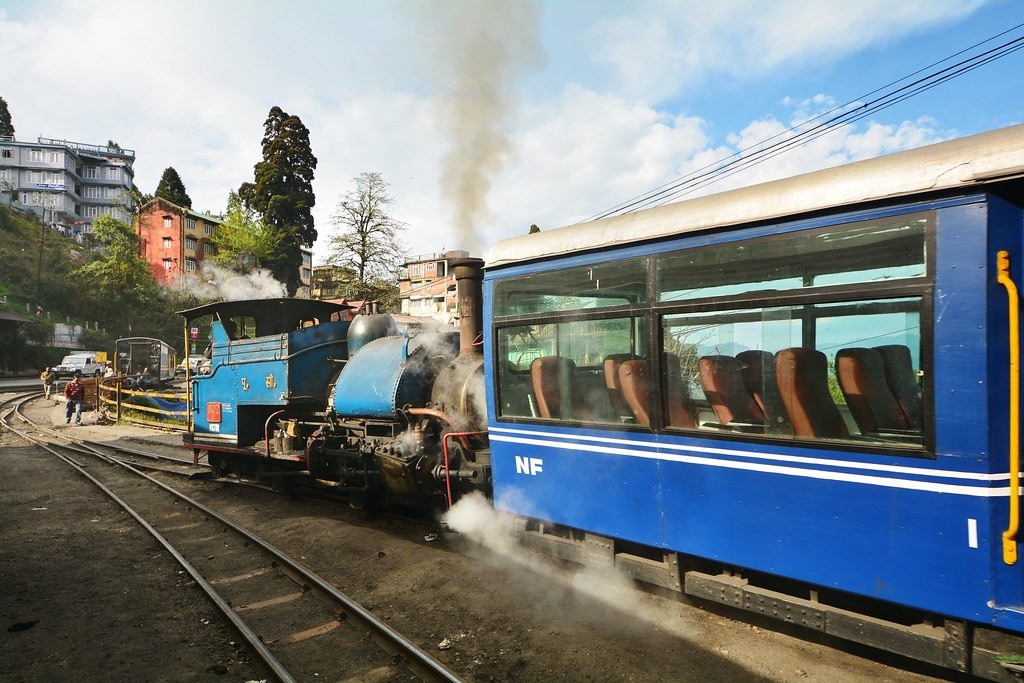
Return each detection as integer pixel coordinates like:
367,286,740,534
64,371,85,425
103,361,114,378
40,366,56,400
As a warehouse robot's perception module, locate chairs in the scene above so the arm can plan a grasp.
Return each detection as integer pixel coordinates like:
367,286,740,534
530,344,922,439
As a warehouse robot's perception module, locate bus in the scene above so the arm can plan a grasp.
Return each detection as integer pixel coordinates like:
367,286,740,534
115,337,178,381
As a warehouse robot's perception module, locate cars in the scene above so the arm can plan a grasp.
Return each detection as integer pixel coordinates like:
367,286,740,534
174,354,208,376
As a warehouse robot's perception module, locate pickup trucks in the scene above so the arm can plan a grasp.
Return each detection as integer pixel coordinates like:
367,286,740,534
51,353,108,380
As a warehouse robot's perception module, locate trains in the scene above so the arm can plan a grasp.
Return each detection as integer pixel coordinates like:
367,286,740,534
182,123,1024,682
